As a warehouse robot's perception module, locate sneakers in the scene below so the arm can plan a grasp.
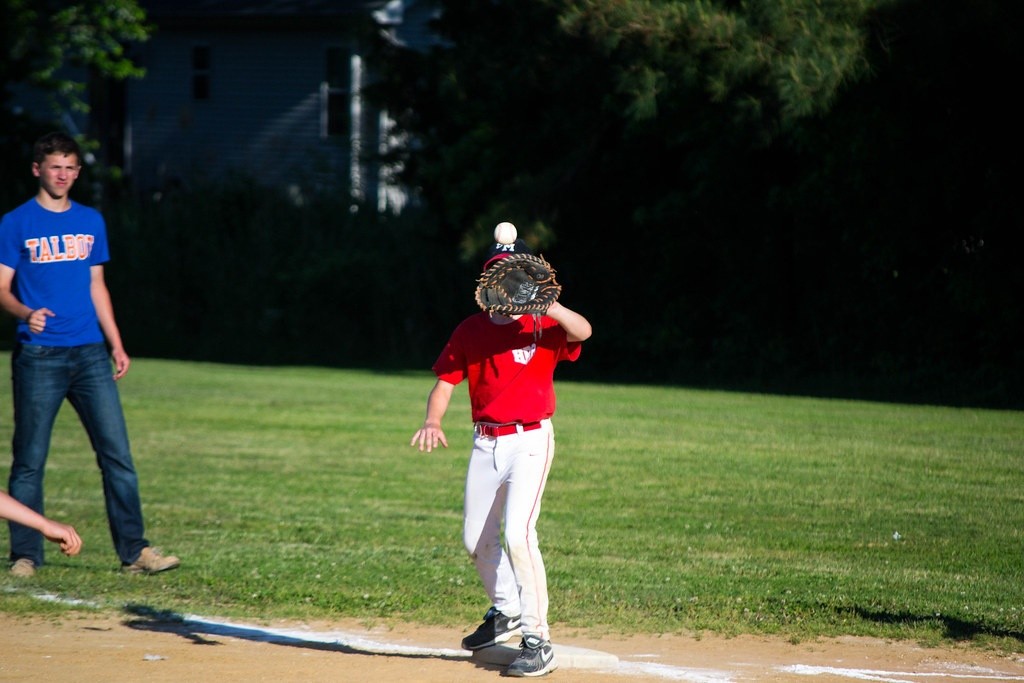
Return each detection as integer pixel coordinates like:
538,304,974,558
461,607,523,651
119,545,181,575
9,557,36,578
504,634,558,676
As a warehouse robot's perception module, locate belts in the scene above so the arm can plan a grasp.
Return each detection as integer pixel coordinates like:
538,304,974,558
473,421,542,438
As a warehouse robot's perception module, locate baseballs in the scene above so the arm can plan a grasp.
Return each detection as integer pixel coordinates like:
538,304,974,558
493,222,518,245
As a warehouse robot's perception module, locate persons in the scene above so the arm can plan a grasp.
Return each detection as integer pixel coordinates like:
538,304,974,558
0,134,181,578
409,239,592,677
0,491,83,556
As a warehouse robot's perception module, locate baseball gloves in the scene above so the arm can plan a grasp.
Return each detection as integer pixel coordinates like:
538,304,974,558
473,252,562,343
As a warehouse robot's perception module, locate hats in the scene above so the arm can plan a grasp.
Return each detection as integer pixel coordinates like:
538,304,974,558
483,239,534,275
